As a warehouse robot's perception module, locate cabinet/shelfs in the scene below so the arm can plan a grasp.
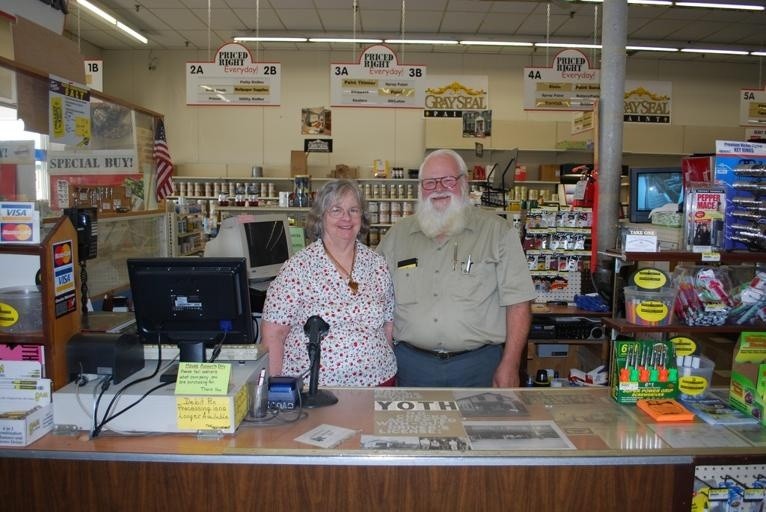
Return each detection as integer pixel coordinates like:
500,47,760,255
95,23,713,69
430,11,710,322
518,200,594,272
0,217,82,393
524,301,608,384
168,201,204,257
599,246,765,333
171,177,559,251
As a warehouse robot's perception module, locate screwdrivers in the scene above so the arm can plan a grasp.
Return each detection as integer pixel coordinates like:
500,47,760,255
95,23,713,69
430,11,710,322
620,343,678,382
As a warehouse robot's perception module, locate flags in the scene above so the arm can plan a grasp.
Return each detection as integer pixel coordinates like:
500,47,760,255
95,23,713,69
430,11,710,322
151,116,177,203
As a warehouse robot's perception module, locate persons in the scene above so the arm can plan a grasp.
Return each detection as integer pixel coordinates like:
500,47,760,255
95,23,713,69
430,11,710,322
374,148,539,388
260,180,399,387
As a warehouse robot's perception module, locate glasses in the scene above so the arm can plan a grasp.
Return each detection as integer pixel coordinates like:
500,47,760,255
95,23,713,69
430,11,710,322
421,174,464,190
327,205,364,218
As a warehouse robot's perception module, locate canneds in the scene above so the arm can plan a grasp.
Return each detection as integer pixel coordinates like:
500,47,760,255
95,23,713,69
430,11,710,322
170,182,276,200
359,183,416,201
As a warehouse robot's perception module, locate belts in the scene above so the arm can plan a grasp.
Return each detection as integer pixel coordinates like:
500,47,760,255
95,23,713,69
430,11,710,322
405,343,489,359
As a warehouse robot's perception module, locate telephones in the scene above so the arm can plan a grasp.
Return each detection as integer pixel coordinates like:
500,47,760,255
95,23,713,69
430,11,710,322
63,207,98,263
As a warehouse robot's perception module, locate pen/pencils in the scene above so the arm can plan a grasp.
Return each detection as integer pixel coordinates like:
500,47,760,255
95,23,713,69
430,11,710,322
254,368,265,417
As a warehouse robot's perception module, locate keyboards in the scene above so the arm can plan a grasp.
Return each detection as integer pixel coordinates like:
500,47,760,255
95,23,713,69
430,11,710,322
144,345,266,360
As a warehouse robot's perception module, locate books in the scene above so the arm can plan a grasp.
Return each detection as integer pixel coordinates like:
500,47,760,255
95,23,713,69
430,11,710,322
636,399,695,422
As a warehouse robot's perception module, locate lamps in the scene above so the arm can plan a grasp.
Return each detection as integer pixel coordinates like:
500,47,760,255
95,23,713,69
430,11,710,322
747,50,766,57
534,43,601,49
458,41,533,47
308,38,384,44
678,48,750,54
627,0,673,6
624,46,680,51
233,37,308,43
673,0,764,13
384,39,458,44
76,0,149,46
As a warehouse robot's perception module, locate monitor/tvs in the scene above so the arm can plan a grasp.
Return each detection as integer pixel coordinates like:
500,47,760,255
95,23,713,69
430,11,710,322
126,257,254,383
204,214,290,283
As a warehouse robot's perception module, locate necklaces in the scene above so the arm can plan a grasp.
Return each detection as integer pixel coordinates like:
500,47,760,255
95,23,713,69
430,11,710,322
321,239,359,295
